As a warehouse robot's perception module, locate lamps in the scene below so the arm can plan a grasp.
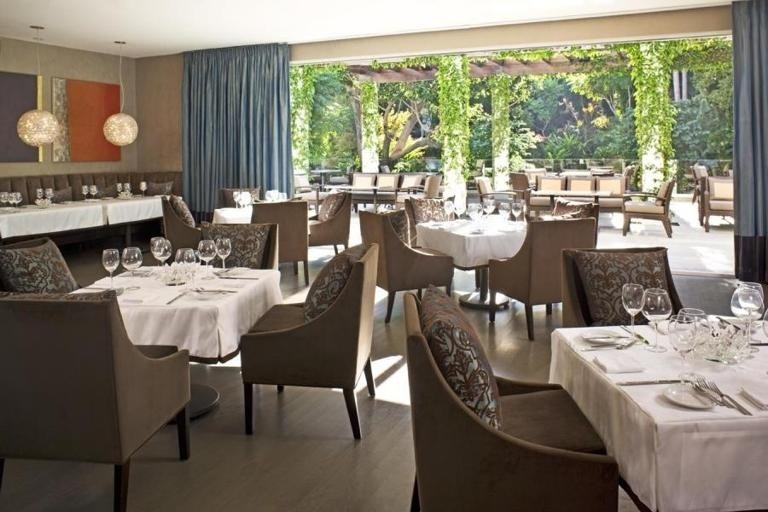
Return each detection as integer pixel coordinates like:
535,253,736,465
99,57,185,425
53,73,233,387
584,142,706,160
17,26,58,146
104,41,139,146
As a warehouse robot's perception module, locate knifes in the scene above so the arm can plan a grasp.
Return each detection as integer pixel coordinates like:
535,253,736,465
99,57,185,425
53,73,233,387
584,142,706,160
218,274,260,280
616,379,691,388
166,290,189,307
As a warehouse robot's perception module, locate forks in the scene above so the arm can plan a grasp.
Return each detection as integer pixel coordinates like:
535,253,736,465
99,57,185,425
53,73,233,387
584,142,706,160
694,378,768,417
590,338,637,349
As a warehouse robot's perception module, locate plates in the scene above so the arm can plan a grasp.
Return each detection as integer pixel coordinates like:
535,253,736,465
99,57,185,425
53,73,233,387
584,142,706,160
661,383,721,410
583,327,631,348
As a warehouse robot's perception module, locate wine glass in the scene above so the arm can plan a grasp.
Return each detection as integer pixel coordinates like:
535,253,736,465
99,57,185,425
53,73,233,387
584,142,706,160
174,238,231,293
231,190,288,213
151,237,172,277
103,248,120,295
444,198,529,234
621,281,768,379
121,246,142,291
0,180,147,213
